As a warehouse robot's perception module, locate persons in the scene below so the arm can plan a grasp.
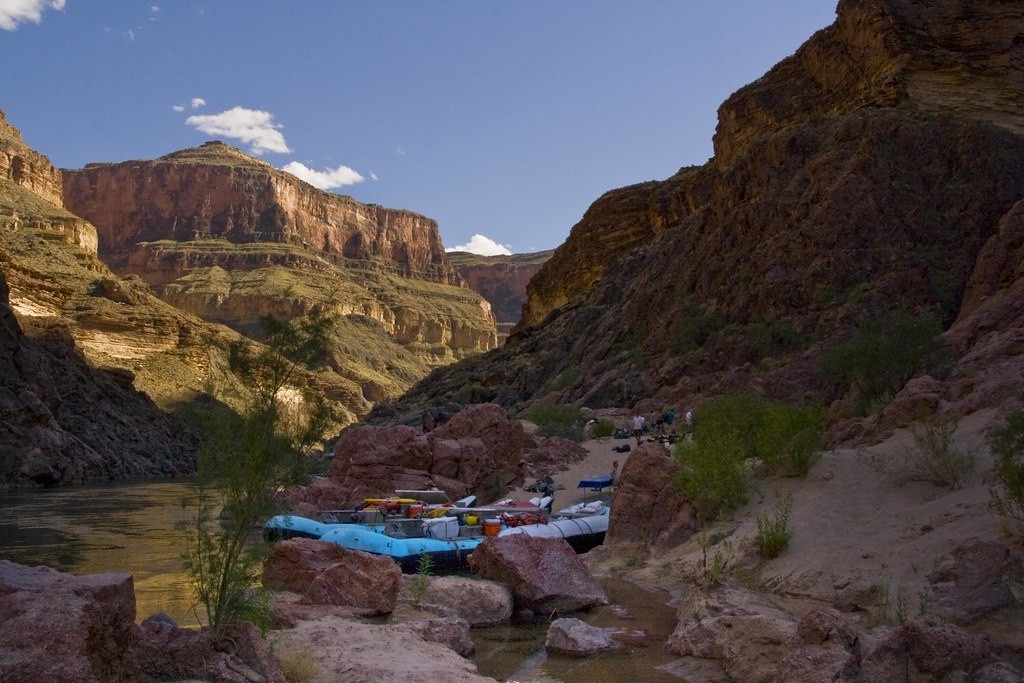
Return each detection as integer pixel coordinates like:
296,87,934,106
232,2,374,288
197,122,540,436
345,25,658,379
611,460,620,494
585,420,594,438
425,480,439,491
650,409,677,436
632,412,645,444
686,409,693,425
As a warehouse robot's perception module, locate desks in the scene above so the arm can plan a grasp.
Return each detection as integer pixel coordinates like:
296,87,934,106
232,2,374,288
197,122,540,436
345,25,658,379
577,475,613,500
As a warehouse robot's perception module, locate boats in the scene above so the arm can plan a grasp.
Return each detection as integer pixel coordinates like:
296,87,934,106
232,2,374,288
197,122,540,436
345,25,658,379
260,489,557,544
317,503,611,580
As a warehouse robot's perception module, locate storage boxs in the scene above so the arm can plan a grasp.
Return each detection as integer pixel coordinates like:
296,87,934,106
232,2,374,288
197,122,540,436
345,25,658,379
459,525,483,537
409,504,422,518
385,519,424,539
423,517,459,539
483,522,500,536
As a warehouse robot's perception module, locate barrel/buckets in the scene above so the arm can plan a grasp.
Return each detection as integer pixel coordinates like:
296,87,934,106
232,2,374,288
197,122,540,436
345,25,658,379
484,522,500,537
410,505,422,519
